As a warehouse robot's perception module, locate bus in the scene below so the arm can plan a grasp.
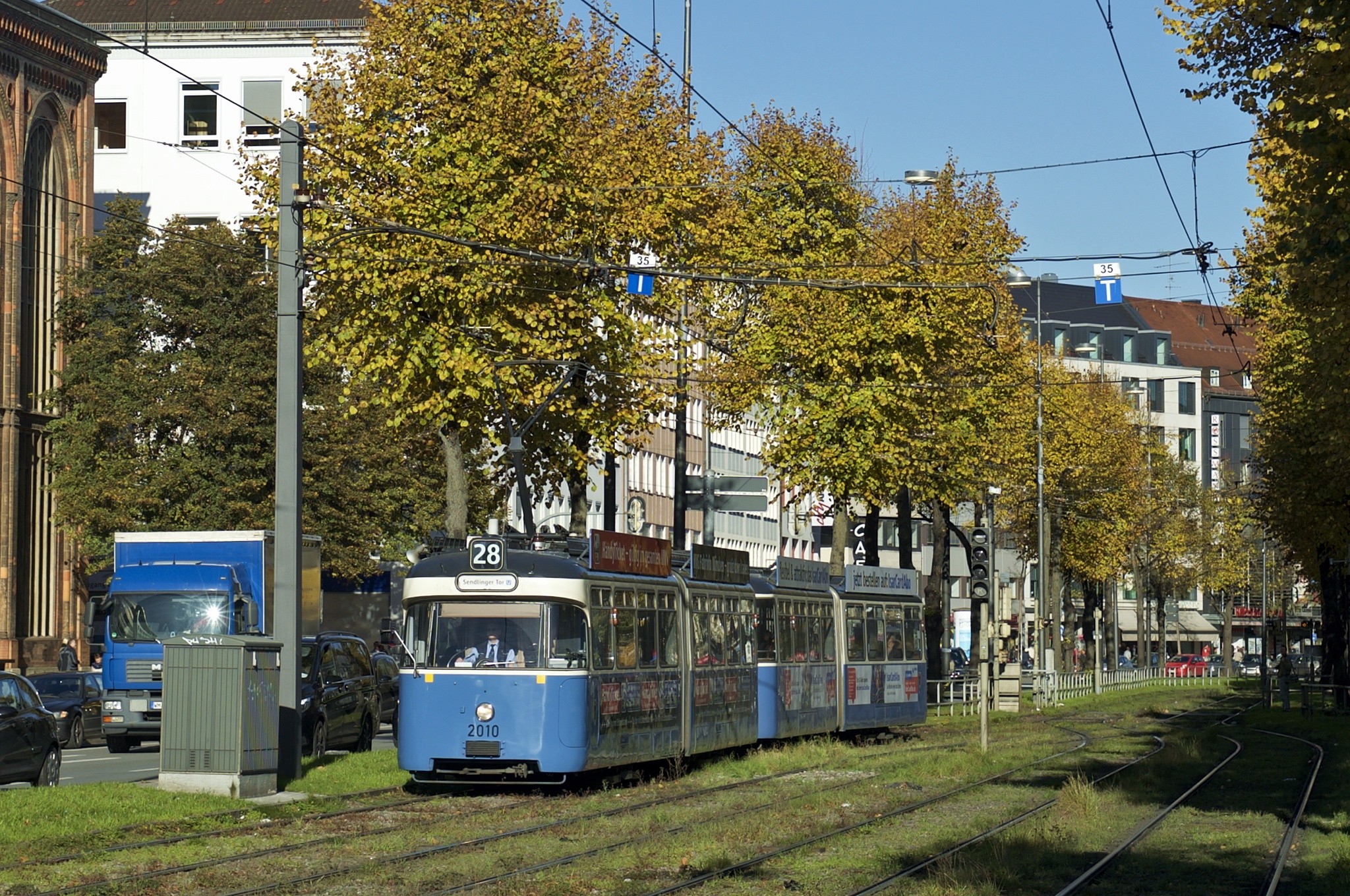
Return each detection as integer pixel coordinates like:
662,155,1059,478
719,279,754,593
380,360,930,791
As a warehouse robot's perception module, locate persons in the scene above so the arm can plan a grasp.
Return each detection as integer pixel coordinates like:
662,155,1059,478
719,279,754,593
457,622,511,665
370,641,388,655
92,653,102,669
758,631,775,650
57,638,81,672
376,645,384,653
1124,647,1131,661
1202,644,1211,662
1130,644,1138,659
1235,647,1245,661
1118,643,1124,655
1274,647,1291,712
1154,644,1158,650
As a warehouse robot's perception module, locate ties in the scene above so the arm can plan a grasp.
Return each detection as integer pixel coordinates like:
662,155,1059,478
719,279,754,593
488,645,495,660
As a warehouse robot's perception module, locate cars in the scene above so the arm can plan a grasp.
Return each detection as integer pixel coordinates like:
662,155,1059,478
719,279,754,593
951,646,970,679
26,671,104,749
1267,653,1312,676
1165,653,1262,679
0,671,63,788
1099,651,1169,673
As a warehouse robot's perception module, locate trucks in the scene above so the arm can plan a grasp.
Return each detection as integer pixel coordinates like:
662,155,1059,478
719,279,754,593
83,530,324,753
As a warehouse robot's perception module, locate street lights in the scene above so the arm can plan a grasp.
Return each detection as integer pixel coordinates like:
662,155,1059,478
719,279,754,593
1003,275,1047,675
1125,385,1151,668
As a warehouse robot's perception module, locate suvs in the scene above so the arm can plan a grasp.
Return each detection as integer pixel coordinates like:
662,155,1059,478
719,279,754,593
299,631,400,758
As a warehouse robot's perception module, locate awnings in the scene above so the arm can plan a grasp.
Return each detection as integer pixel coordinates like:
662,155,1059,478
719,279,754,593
1118,609,1219,641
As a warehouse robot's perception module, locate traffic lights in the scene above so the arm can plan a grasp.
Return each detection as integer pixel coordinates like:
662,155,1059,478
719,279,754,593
968,525,991,601
